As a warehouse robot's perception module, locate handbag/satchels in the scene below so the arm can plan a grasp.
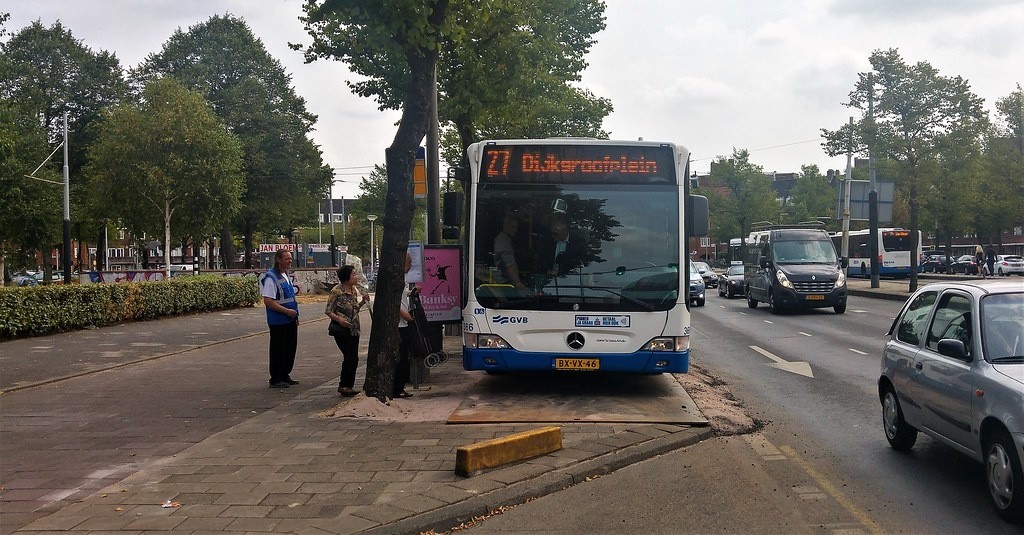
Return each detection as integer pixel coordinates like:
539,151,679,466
327,320,352,336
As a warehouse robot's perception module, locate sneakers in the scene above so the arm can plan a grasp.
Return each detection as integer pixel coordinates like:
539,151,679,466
337,386,361,397
288,376,299,385
393,389,413,398
269,378,289,388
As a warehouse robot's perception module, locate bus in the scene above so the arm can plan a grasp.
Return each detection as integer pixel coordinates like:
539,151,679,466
827,228,923,279
443,138,709,381
983,243,1024,258
931,245,981,261
729,237,750,266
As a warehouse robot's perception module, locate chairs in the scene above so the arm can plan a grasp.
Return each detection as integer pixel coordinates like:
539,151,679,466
610,238,654,286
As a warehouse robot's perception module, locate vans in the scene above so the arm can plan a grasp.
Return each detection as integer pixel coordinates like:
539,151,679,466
746,221,848,314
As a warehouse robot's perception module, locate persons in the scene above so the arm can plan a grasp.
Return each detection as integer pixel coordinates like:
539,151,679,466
490,216,528,292
984,244,998,277
324,265,370,397
603,239,644,276
541,217,591,276
396,253,423,399
261,248,301,388
975,244,985,276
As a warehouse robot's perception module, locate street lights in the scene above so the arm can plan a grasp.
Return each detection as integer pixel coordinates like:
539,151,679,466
329,179,346,266
367,215,378,279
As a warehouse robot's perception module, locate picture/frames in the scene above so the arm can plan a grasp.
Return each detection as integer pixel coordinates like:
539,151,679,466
409,244,463,325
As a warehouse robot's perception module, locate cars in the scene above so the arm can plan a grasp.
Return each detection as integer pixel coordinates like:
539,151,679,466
693,262,718,288
878,280,1024,522
923,255,955,273
951,255,979,275
718,263,746,298
984,255,1023,276
689,259,706,307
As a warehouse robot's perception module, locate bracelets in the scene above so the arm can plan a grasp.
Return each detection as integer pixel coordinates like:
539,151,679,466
335,315,339,322
